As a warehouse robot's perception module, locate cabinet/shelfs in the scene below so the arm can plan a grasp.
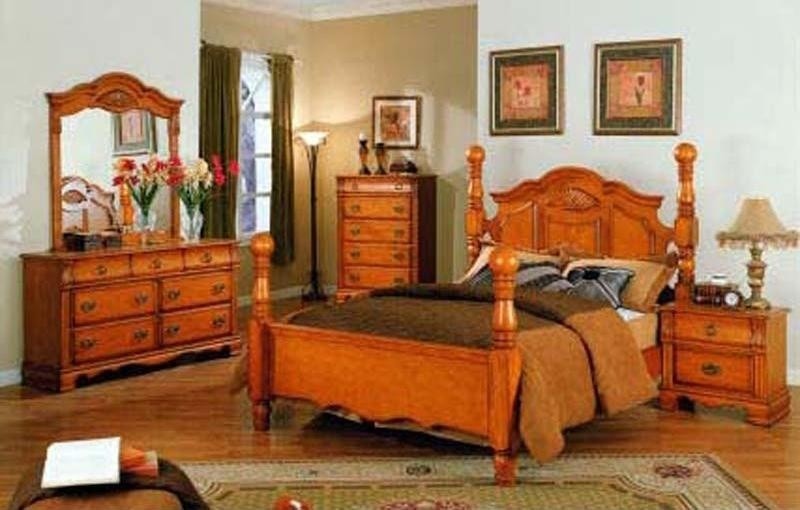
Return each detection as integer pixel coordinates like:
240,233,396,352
19,231,243,391
328,169,436,302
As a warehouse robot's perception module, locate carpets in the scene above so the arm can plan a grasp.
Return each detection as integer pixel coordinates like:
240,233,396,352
167,448,777,507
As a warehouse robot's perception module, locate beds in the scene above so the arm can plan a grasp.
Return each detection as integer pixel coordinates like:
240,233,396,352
243,139,700,487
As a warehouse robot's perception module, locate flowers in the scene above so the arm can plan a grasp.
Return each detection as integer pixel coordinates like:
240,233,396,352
165,150,240,242
113,152,169,225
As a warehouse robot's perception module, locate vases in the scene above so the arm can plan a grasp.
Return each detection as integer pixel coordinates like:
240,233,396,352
132,204,155,234
178,203,204,244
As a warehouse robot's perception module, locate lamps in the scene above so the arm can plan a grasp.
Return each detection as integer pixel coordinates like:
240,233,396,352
294,127,331,304
717,192,800,312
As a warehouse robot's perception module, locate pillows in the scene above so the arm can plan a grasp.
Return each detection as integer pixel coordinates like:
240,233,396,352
460,231,674,312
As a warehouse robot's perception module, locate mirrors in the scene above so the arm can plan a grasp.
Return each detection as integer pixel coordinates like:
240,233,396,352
46,66,187,248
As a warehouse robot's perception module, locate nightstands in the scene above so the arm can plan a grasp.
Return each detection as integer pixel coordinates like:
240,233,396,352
652,297,794,427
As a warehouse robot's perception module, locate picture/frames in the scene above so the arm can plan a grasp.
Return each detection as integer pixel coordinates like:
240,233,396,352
489,44,567,138
109,107,159,158
371,95,422,151
590,37,684,137
